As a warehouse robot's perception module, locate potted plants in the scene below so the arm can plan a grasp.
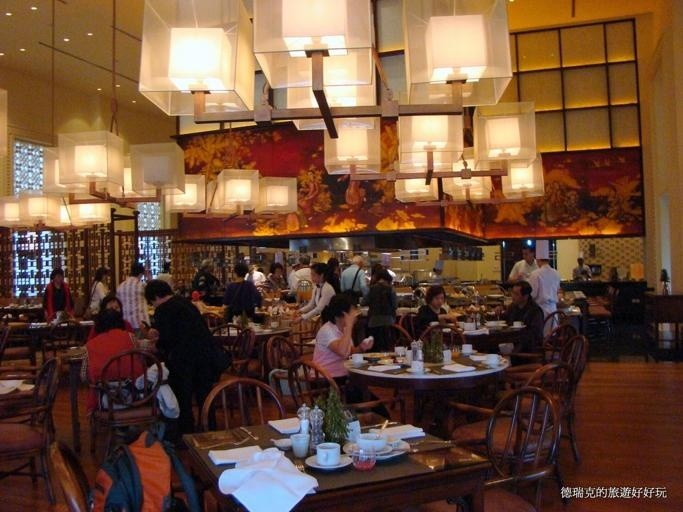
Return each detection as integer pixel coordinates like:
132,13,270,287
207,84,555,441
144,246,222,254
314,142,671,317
658,269,671,295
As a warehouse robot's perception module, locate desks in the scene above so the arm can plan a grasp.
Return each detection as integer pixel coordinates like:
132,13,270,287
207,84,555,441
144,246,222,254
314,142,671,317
645,291,683,363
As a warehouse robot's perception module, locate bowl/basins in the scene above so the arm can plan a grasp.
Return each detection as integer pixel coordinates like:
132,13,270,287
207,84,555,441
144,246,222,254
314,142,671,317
484,320,507,331
357,432,388,451
275,438,292,451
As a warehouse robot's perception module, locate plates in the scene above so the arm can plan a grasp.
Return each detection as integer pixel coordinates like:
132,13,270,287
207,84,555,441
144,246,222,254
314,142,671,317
348,360,368,369
304,454,353,472
342,439,410,461
510,325,527,329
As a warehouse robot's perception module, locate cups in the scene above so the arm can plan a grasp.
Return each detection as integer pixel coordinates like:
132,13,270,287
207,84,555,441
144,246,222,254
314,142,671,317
462,344,473,352
394,346,424,371
513,321,524,327
451,345,460,359
458,321,475,331
316,442,341,467
352,445,376,471
349,353,364,362
271,322,279,329
486,354,502,362
290,433,310,458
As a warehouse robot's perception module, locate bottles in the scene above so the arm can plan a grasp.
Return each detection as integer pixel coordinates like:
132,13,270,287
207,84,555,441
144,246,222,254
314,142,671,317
411,338,424,361
297,403,326,449
470,312,481,329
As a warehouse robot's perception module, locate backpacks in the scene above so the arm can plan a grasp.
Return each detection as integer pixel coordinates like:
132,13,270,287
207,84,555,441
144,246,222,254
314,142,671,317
90,418,202,511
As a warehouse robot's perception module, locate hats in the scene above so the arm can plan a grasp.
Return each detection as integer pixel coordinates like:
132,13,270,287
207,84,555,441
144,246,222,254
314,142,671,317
381,252,391,267
274,253,282,266
434,260,444,270
536,239,550,259
579,252,584,259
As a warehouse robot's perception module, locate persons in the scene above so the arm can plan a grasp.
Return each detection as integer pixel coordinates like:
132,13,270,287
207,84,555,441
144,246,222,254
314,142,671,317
145,280,218,449
80,309,143,431
414,287,464,340
310,295,391,421
430,264,443,285
494,281,543,366
572,255,591,281
508,246,539,285
43,255,396,353
529,250,561,337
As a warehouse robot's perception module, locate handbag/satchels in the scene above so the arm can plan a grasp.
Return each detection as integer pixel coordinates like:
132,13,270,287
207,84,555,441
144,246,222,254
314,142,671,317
82,307,91,321
204,335,231,370
223,304,235,323
351,290,362,304
96,378,135,412
